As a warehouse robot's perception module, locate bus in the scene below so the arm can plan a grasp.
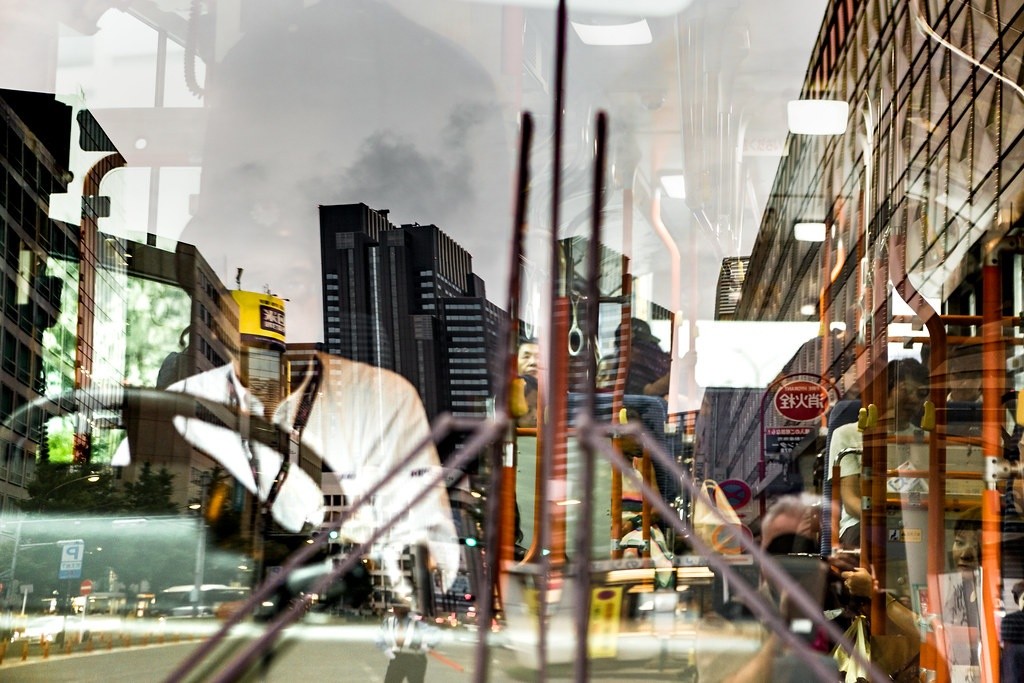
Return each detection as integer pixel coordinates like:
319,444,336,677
154,584,250,620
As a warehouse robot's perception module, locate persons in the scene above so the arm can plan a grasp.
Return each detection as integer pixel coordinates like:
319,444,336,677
109,195,463,605
696,433,1024,683
827,340,1023,614
376,598,443,683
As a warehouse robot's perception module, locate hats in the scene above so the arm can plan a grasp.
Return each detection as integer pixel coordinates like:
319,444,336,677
616,318,661,346
390,598,409,608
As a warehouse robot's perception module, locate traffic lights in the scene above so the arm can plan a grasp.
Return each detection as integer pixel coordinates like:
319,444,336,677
459,538,477,547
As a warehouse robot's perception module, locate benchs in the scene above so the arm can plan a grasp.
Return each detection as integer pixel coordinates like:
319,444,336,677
566,391,667,528
820,393,983,552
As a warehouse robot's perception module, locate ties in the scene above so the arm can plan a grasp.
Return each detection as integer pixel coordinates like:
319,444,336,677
395,621,404,647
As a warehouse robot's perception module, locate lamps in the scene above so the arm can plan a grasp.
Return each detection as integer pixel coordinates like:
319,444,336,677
785,90,849,137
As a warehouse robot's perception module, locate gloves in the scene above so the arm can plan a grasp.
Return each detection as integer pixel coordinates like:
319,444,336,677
421,643,431,652
385,649,395,659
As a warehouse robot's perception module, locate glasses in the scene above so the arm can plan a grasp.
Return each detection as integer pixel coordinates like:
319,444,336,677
898,383,930,398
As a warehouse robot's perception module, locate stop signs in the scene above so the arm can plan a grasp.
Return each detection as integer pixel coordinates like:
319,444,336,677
79,579,92,594
